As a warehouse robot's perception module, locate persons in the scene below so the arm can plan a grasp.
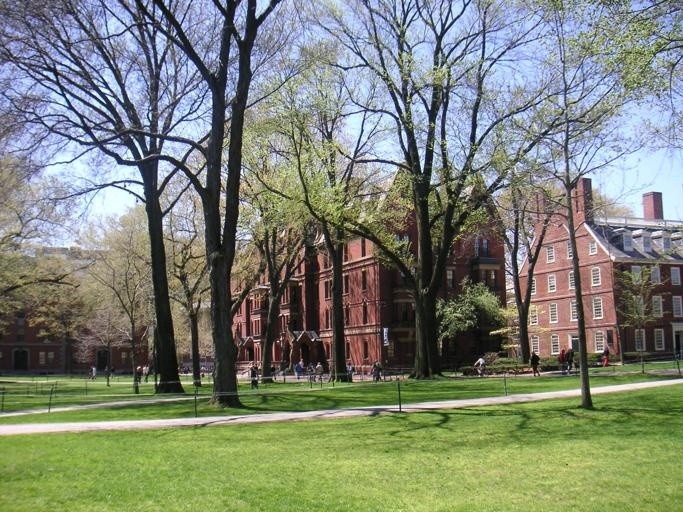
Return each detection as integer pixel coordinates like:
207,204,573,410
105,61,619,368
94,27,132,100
530,351,541,377
271,364,276,380
87,364,150,384
564,348,574,376
599,344,609,368
294,357,384,382
240,360,258,390
558,348,567,376
473,354,485,376
